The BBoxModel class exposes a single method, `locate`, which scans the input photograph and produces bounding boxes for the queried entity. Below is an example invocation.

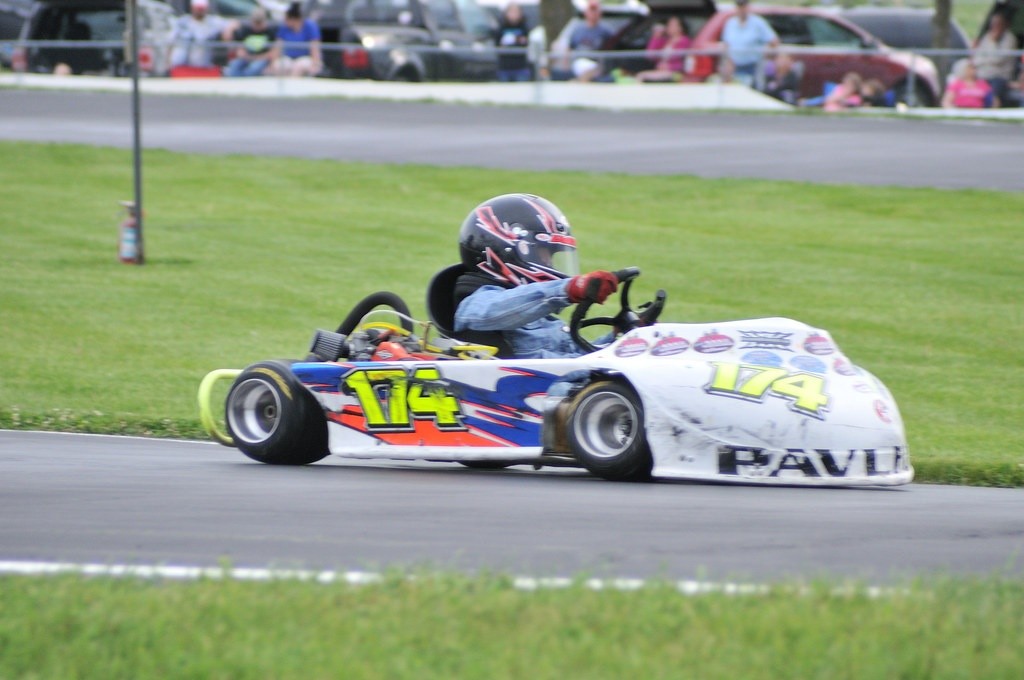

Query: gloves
[565,271,618,304]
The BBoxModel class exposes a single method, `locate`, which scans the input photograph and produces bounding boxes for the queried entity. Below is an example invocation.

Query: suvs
[842,9,973,88]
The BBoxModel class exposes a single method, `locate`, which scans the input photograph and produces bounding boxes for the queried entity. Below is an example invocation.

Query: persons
[223,11,283,76]
[822,71,898,112]
[938,15,1018,109]
[267,7,325,79]
[171,0,234,68]
[489,1,535,82]
[706,0,806,106]
[568,0,617,83]
[645,13,694,73]
[443,193,618,360]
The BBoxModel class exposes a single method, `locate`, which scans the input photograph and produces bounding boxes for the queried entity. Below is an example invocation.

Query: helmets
[458,193,580,287]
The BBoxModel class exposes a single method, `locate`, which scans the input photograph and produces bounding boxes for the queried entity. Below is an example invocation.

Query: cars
[549,0,718,82]
[0,0,273,76]
[319,0,503,83]
[687,5,944,106]
